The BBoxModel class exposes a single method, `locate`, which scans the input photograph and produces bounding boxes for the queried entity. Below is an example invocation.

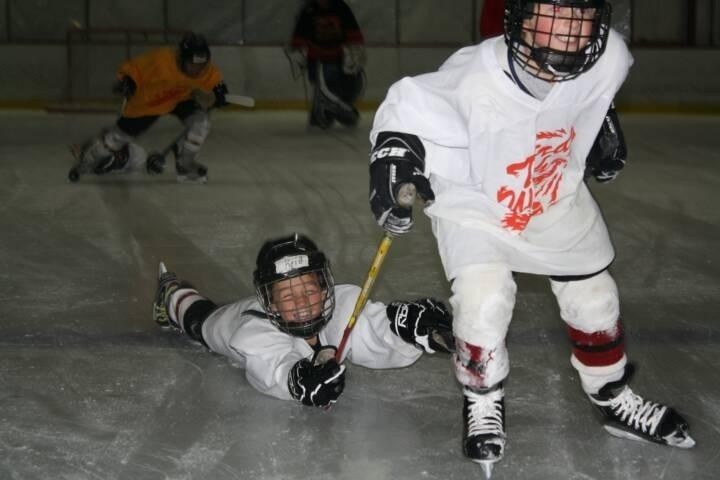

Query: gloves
[384,296,457,356]
[210,82,232,110]
[586,103,629,185]
[365,131,438,237]
[112,74,137,98]
[286,345,348,410]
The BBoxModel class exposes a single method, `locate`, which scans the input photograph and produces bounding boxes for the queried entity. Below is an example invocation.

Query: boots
[174,146,209,178]
[80,139,104,176]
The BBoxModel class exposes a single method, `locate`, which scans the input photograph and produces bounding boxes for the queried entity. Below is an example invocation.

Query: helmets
[249,230,333,309]
[176,29,212,70]
[501,0,611,61]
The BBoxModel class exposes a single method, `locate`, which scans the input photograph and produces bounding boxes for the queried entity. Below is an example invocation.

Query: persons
[69,32,229,185]
[364,0,693,467]
[281,0,369,131]
[151,230,454,410]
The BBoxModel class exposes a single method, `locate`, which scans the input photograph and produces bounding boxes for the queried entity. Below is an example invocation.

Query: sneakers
[582,362,691,447]
[458,373,510,462]
[152,271,180,328]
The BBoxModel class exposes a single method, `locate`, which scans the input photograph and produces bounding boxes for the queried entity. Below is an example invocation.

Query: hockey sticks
[335,184,415,363]
[161,93,255,159]
